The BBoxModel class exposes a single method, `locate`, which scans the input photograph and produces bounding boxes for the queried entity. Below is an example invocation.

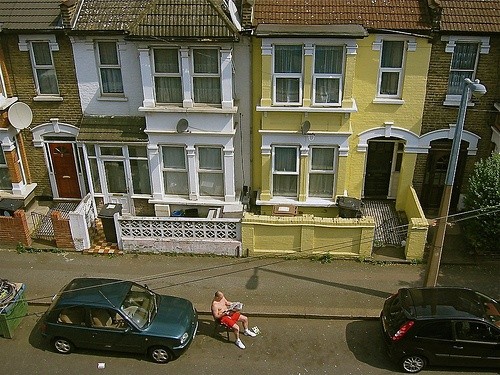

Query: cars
[38,277,200,365]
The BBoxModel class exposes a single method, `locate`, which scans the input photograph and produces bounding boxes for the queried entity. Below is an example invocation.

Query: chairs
[59,306,84,324]
[212,311,231,341]
[92,307,125,329]
[107,306,138,323]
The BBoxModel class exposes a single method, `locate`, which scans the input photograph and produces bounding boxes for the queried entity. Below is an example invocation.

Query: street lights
[422,77,487,288]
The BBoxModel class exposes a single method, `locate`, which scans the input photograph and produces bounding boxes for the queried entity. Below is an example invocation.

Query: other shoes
[235,340,246,349]
[244,330,256,337]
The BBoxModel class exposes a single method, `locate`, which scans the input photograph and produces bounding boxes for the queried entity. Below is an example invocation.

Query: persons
[211,291,257,350]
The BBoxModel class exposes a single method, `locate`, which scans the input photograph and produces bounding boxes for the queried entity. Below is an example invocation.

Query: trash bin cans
[336,196,364,218]
[0,198,24,217]
[97,202,123,244]
[272,204,298,217]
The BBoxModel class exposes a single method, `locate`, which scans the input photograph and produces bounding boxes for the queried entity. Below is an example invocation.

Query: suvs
[378,285,500,374]
